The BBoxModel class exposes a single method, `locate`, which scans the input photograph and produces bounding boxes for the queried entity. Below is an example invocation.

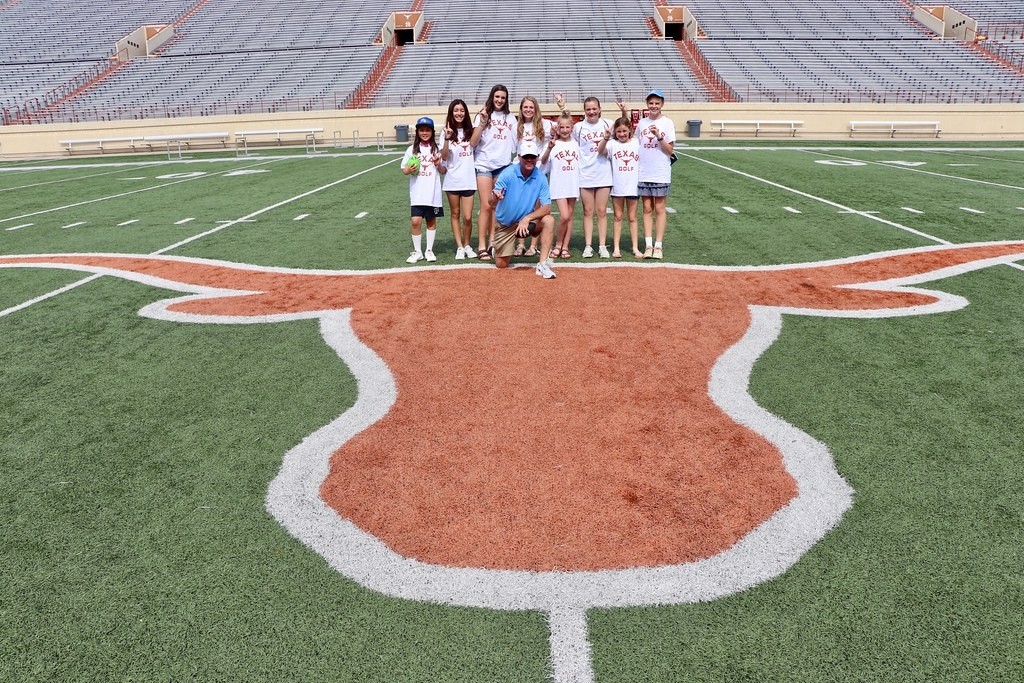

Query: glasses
[522,155,537,159]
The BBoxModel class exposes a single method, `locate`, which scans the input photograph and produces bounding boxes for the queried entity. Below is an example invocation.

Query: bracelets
[560,103,568,112]
[657,137,663,141]
[479,120,487,128]
[445,138,451,141]
[435,163,441,167]
[604,138,608,141]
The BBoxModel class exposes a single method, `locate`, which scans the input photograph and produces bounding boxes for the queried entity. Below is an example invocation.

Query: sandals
[549,247,572,259]
[514,244,525,256]
[477,246,493,261]
[524,247,538,257]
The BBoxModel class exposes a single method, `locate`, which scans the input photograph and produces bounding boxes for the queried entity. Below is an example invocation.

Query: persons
[550,96,628,258]
[541,114,581,258]
[634,90,676,259]
[512,91,569,256]
[487,141,558,279]
[400,117,448,264]
[436,99,478,260]
[597,116,675,259]
[470,84,519,261]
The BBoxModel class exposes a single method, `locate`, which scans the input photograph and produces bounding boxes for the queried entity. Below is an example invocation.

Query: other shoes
[633,250,643,259]
[612,251,621,257]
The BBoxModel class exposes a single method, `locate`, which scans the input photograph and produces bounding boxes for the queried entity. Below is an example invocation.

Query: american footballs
[515,222,536,238]
[408,155,420,175]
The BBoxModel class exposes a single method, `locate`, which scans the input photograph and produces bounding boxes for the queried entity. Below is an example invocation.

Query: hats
[415,117,434,128]
[518,141,538,157]
[645,89,664,99]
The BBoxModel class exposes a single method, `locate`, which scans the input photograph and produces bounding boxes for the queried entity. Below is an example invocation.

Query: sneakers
[405,250,424,264]
[598,246,610,259]
[424,250,436,262]
[535,261,556,279]
[582,246,594,258]
[643,246,663,260]
[454,245,477,259]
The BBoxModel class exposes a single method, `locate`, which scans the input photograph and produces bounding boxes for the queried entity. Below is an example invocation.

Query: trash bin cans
[687,120,702,137]
[394,124,409,142]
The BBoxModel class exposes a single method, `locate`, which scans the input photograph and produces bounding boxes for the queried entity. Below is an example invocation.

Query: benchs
[0,0,1024,157]
